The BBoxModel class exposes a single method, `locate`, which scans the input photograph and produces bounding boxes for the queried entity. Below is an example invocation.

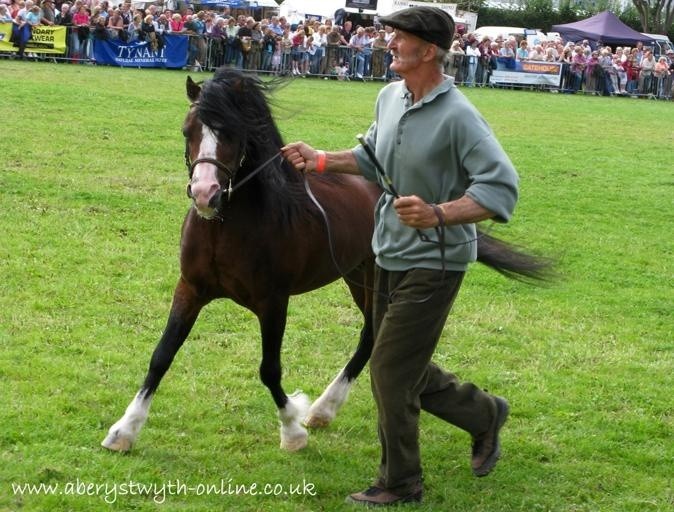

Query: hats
[378,7,456,51]
[665,50,673,55]
[568,41,574,47]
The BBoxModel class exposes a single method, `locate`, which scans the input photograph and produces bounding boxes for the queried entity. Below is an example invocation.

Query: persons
[280,6,521,505]
[0,0,674,99]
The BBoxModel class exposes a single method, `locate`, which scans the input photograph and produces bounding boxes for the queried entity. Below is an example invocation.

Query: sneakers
[292,68,300,76]
[471,393,507,477]
[615,88,627,94]
[345,485,423,504]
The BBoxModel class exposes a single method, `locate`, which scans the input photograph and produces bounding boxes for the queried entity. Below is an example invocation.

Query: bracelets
[315,149,325,174]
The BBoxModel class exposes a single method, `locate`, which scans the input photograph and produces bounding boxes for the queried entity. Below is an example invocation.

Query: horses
[100,61,558,455]
